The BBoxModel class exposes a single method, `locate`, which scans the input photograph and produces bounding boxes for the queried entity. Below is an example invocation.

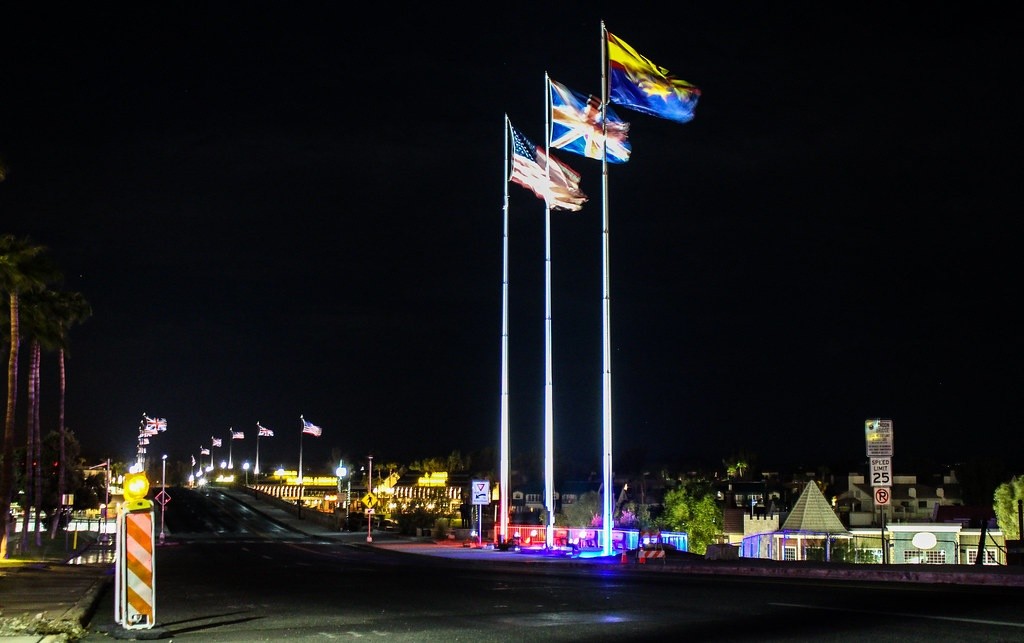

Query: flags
[191,457,196,466]
[550,78,631,164]
[258,425,274,436]
[213,438,222,447]
[302,418,322,436]
[508,118,589,212]
[201,449,210,455]
[607,31,700,124]
[138,417,166,463]
[232,432,244,439]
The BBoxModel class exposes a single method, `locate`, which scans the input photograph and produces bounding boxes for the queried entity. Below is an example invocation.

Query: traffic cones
[621,544,629,564]
[639,547,646,565]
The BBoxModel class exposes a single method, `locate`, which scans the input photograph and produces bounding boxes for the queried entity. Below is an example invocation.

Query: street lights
[243,462,250,486]
[278,468,285,499]
